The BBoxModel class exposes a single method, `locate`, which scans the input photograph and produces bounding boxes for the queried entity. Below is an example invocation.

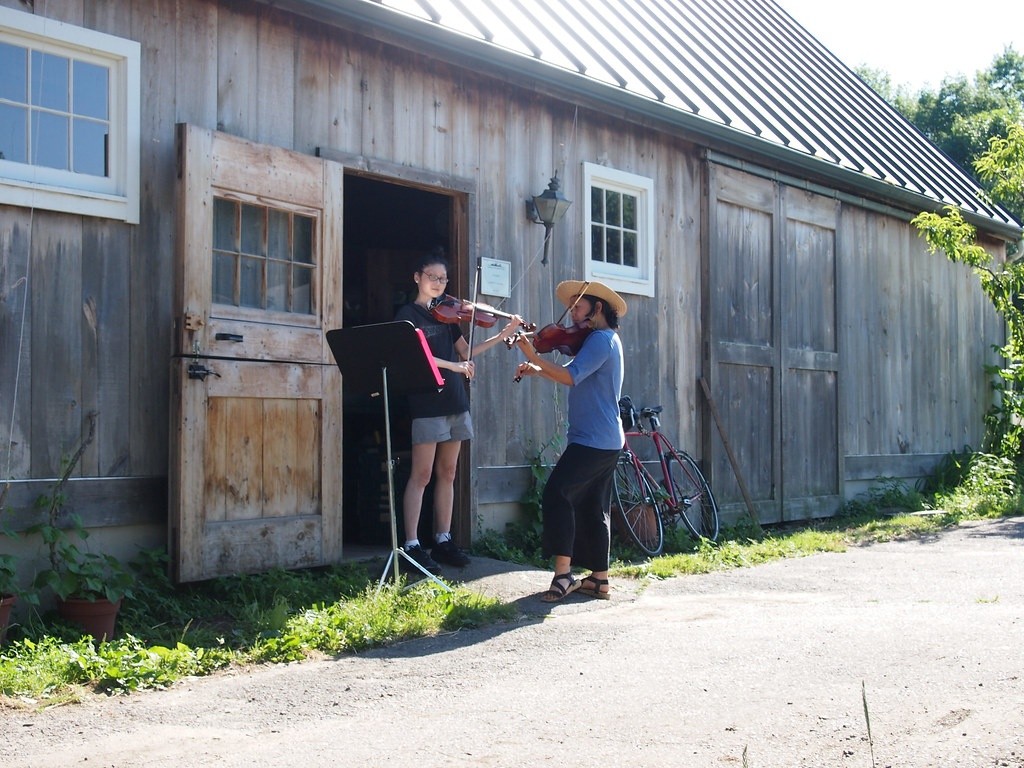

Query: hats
[556,280,627,318]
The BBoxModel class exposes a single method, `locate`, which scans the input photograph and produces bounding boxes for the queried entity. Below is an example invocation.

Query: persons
[514,279,627,605]
[392,253,524,571]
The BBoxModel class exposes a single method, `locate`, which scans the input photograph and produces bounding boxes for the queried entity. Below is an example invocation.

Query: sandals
[574,576,611,599]
[540,572,581,602]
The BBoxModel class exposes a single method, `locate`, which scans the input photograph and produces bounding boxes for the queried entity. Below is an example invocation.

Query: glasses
[421,270,449,284]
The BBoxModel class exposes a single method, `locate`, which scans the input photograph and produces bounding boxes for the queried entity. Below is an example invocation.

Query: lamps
[526,168,572,269]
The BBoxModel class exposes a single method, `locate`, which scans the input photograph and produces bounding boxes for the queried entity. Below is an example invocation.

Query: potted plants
[1,408,128,653]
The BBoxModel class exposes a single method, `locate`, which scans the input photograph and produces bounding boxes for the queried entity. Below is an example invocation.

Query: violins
[432,292,536,333]
[503,321,595,357]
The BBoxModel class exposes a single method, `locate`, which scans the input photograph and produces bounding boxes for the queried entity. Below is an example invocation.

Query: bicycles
[611,395,720,557]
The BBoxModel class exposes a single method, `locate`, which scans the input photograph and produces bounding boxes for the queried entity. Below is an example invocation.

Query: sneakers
[400,545,441,575]
[430,541,471,566]
